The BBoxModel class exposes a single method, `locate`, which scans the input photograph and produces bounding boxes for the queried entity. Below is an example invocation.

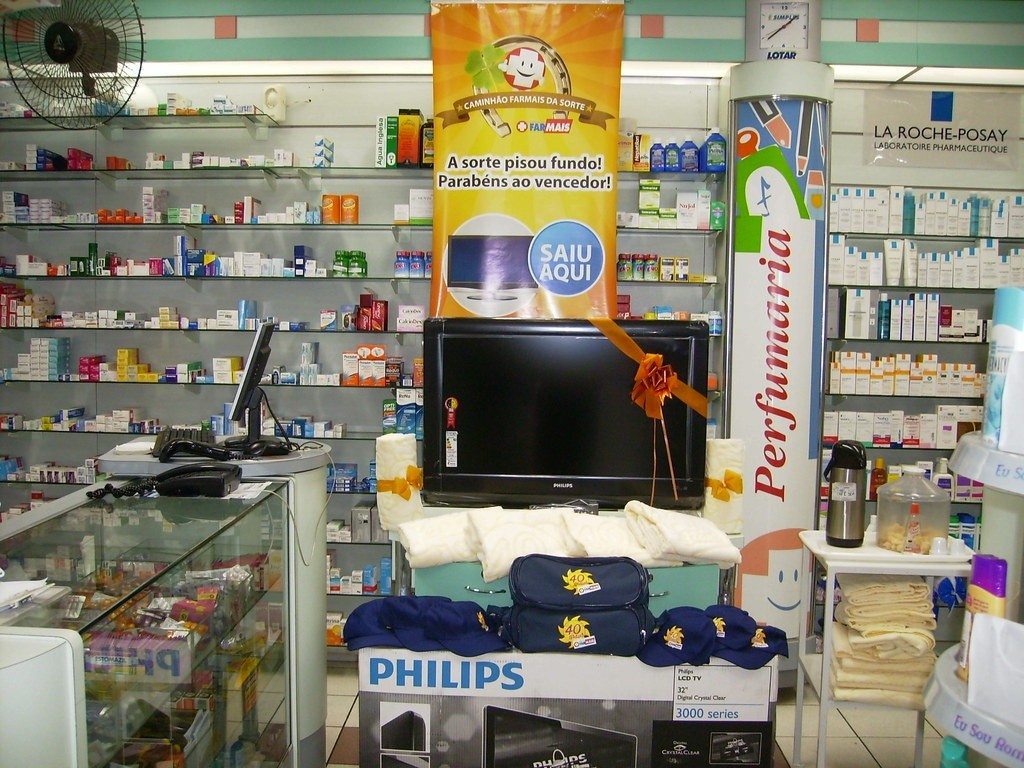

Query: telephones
[154,462,243,497]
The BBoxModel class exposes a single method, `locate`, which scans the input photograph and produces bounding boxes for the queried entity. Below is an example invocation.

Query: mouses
[243,441,289,457]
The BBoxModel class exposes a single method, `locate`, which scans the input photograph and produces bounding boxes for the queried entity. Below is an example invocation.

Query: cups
[929,537,965,556]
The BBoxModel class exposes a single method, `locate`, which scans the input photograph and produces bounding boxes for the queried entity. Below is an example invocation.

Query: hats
[705,604,757,651]
[636,606,715,667]
[711,626,790,670]
[343,598,404,651]
[425,600,512,657]
[381,595,452,652]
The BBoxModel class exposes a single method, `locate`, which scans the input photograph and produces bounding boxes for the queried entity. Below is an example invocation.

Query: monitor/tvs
[225,322,283,450]
[423,318,712,509]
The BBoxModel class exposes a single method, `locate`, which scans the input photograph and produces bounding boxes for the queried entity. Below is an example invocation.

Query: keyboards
[153,428,215,456]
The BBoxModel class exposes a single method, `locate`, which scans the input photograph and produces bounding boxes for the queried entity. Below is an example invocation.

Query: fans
[1,0,143,130]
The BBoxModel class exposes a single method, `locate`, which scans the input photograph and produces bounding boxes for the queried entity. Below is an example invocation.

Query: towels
[830,572,938,711]
[464,508,576,585]
[624,500,742,571]
[561,510,685,568]
[702,438,744,535]
[397,504,506,570]
[374,432,423,530]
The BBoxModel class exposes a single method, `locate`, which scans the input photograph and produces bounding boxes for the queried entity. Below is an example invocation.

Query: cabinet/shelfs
[1,112,436,662]
[793,527,978,767]
[822,230,1024,505]
[613,170,731,439]
[924,433,1024,768]
[1,477,295,767]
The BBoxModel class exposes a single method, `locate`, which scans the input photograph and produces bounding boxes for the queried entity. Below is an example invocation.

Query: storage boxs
[617,133,717,438]
[821,185,1024,500]
[2,105,434,645]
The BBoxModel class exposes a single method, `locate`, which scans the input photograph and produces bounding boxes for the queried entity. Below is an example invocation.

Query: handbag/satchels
[505,603,656,657]
[509,554,655,610]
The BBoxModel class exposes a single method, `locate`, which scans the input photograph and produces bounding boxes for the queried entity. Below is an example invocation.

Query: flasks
[823,439,867,549]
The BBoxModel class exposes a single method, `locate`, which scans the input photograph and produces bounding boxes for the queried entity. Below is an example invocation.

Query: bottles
[878,187,992,339]
[869,458,888,501]
[708,310,722,337]
[331,249,432,278]
[420,117,435,168]
[649,126,727,173]
[866,514,877,544]
[616,253,659,281]
[904,502,922,554]
[88,242,98,276]
[876,463,950,555]
[933,457,955,502]
